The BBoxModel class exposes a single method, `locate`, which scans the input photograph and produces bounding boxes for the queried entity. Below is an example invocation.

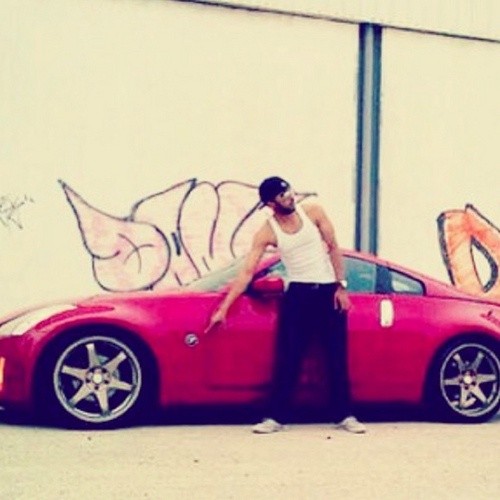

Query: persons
[204,176,366,434]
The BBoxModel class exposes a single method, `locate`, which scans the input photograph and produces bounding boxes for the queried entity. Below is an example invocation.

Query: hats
[258,176,289,206]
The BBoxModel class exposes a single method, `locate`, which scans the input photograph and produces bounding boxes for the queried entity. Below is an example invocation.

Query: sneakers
[252,418,282,433]
[337,416,366,433]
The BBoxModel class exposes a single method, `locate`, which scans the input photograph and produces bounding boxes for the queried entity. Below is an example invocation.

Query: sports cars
[0,240,500,429]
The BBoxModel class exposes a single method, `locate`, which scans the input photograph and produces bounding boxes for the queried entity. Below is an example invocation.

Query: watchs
[338,279,348,288]
